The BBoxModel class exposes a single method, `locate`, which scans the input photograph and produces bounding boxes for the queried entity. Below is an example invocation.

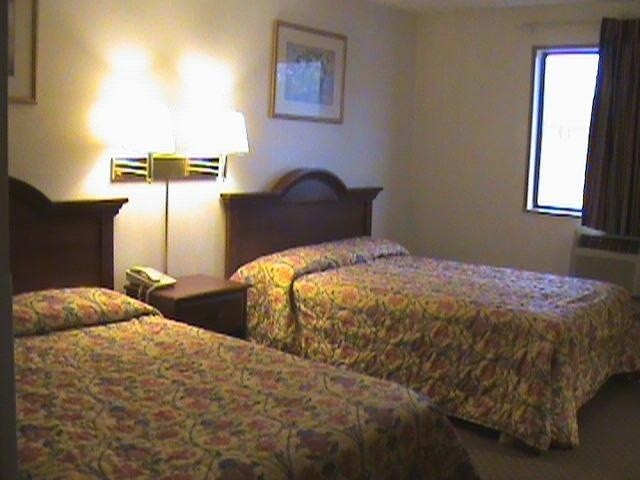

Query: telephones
[126,265,177,289]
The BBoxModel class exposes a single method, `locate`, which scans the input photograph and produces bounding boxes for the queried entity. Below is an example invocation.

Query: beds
[219,168,640,453]
[8,172,471,479]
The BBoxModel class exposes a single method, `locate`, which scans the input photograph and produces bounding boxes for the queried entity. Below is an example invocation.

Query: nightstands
[124,273,253,339]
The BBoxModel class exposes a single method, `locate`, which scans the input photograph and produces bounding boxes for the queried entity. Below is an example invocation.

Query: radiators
[568,227,640,299]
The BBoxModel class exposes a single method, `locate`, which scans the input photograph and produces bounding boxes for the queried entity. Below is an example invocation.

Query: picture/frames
[0,0,40,107]
[269,19,348,125]
